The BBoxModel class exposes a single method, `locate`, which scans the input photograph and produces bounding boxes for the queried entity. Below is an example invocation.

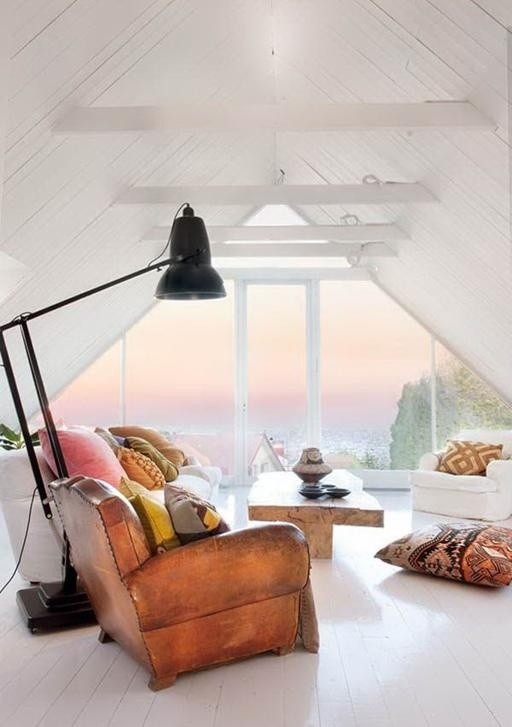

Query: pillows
[37,426,188,487]
[439,439,503,475]
[117,476,183,555]
[374,522,512,587]
[163,483,229,544]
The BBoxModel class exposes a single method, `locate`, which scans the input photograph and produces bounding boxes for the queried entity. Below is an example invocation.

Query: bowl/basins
[298,484,351,499]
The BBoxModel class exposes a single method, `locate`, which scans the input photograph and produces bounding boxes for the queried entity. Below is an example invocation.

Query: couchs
[0,431,222,584]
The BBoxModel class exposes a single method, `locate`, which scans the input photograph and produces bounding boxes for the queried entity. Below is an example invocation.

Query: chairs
[411,429,512,522]
[46,475,319,692]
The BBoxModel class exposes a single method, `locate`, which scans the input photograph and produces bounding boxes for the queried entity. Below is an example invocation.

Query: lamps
[0,201,226,631]
